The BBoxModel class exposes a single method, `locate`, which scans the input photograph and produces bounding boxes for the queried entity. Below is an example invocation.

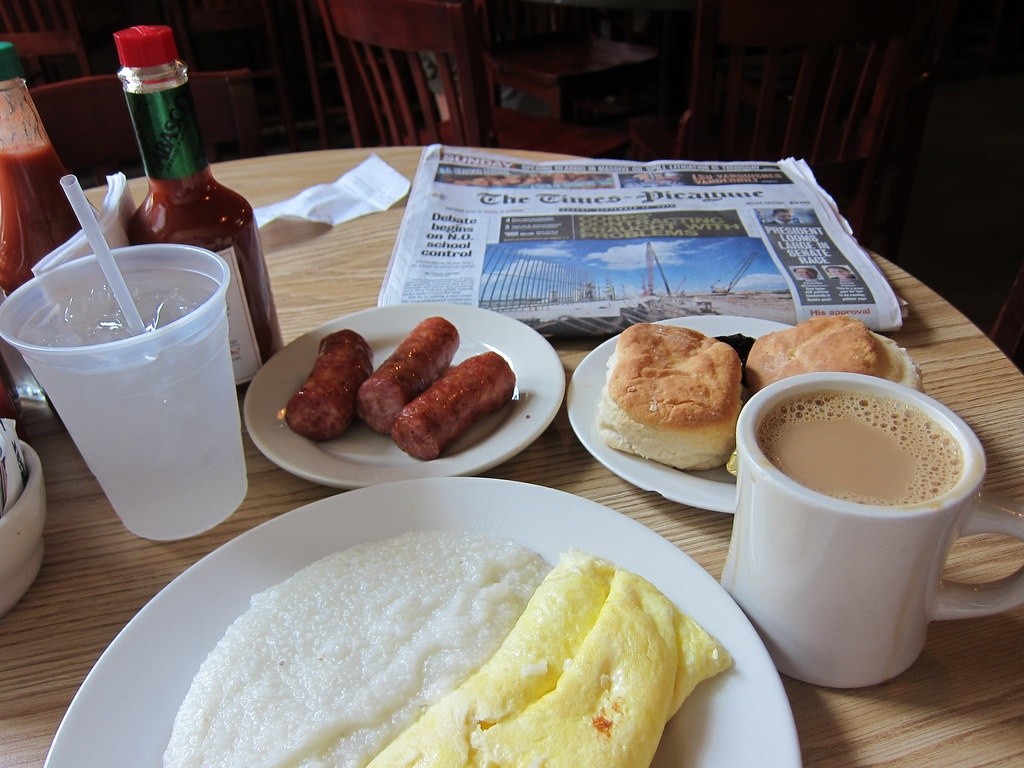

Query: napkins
[32,173,133,276]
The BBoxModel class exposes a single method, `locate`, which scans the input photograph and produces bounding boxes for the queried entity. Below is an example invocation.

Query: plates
[244,302,566,489]
[45,477,804,768]
[566,315,801,513]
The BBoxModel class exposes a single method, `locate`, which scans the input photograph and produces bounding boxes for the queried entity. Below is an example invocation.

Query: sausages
[284,329,374,443]
[354,316,461,434]
[392,351,516,460]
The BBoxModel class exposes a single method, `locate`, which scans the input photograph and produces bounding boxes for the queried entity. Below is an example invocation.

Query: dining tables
[0,149,1024,768]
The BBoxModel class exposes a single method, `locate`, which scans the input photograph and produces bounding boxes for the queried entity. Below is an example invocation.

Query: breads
[742,314,922,396]
[596,322,743,471]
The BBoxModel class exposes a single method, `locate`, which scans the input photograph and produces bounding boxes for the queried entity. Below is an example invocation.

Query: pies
[362,551,734,768]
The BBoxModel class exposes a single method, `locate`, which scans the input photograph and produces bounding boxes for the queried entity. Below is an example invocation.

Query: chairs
[0,0,946,266]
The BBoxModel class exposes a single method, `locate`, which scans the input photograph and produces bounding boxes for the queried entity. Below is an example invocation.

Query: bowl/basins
[0,438,47,618]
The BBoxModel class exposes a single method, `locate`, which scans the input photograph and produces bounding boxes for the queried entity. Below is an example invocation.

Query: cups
[1,242,250,540]
[720,373,1024,688]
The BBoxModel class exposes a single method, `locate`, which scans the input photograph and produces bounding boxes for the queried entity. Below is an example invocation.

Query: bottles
[0,41,107,423]
[114,25,284,399]
